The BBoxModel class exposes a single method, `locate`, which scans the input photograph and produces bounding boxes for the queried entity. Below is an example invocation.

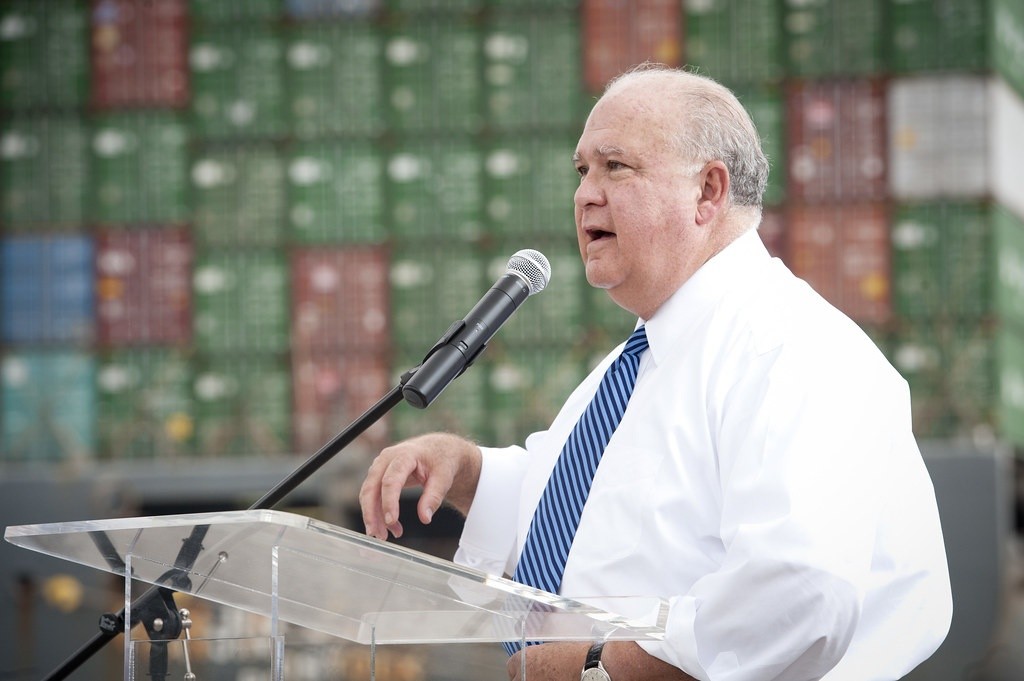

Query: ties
[501,327,649,658]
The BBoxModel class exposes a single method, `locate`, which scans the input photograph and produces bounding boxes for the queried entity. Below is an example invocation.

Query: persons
[359,61,958,680]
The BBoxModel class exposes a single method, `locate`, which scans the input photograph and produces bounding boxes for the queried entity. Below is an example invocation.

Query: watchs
[580,636,614,680]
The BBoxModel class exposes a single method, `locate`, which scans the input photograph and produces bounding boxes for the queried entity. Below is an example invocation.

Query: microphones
[400,249,553,409]
